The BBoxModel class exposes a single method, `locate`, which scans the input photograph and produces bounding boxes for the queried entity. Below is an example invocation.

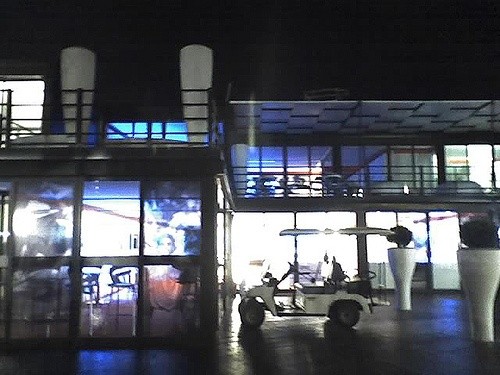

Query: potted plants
[458,218,500,343]
[386,225,417,311]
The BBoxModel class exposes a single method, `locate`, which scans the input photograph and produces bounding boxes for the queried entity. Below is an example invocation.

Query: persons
[320,254,346,282]
[260,256,270,278]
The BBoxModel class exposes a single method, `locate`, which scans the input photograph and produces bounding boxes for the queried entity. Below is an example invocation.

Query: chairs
[82,273,103,330]
[108,264,141,330]
